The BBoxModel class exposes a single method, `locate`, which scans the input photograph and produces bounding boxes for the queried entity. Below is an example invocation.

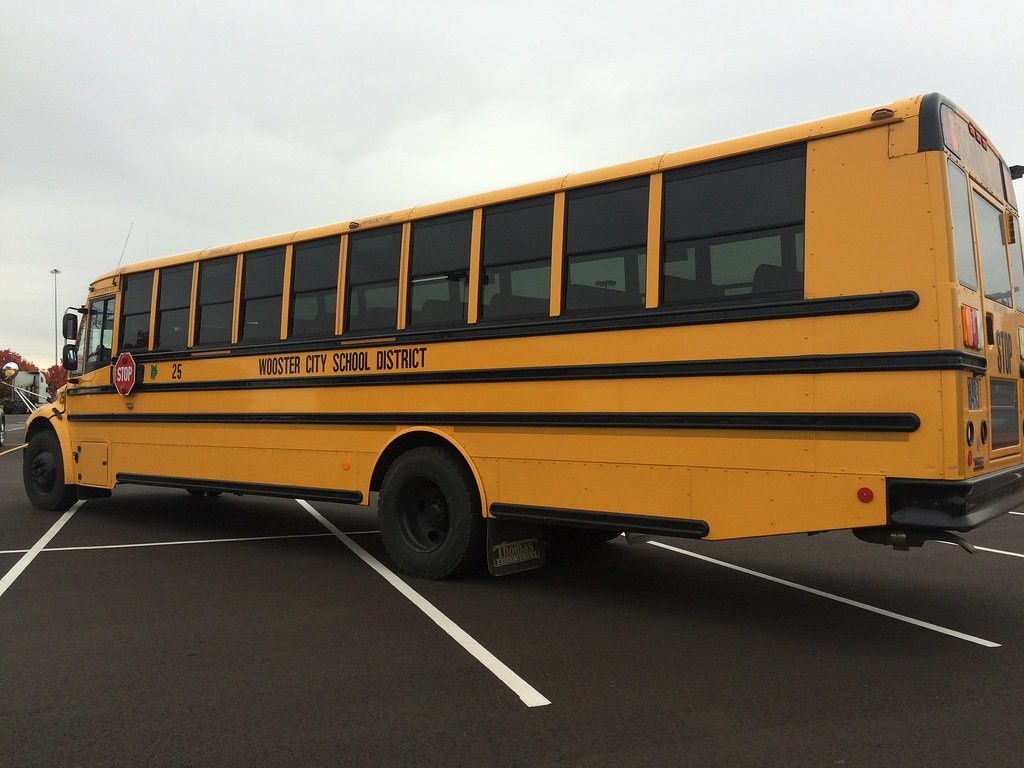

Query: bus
[0,93,1024,584]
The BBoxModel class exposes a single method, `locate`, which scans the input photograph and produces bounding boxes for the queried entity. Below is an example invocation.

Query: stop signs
[113,352,136,396]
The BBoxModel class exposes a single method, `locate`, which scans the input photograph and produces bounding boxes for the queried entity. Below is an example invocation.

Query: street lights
[595,279,616,289]
[50,269,61,367]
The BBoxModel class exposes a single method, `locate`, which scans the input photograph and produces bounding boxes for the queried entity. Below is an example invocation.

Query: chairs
[199,265,801,344]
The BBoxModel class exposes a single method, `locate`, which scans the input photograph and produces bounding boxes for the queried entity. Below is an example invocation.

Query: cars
[0,408,5,447]
